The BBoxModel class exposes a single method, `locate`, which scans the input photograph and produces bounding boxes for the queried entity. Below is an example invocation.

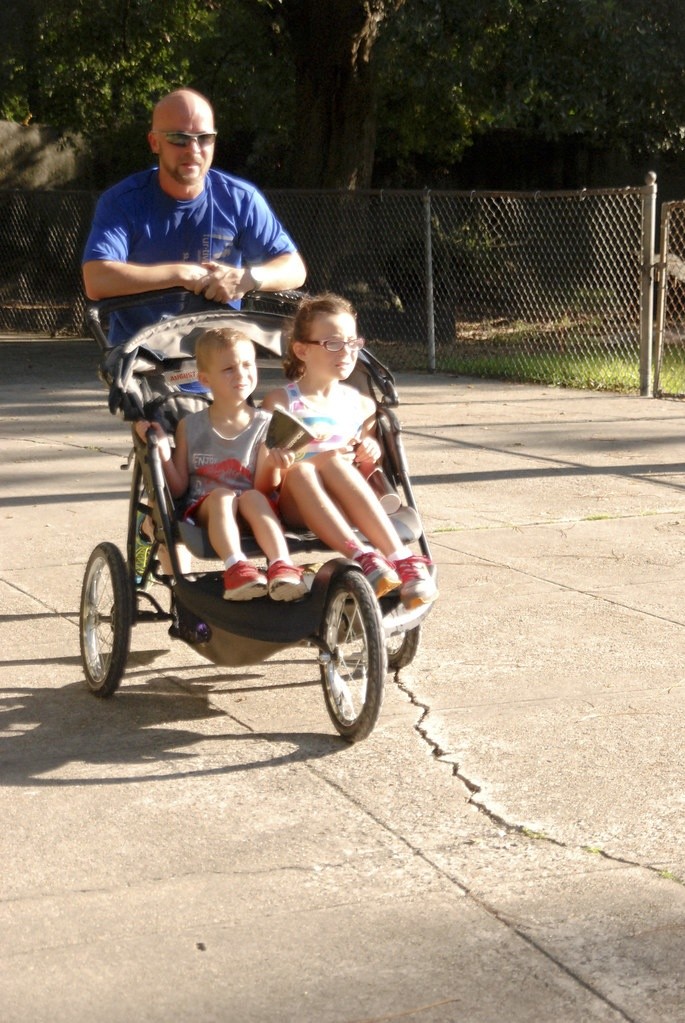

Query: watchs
[249,265,265,293]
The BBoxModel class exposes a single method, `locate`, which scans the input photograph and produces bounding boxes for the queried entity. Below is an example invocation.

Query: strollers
[78,289,432,744]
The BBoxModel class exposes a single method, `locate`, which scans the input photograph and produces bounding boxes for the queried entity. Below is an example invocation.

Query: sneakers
[266,560,309,603]
[221,560,268,601]
[134,515,153,584]
[345,541,402,599]
[392,555,439,610]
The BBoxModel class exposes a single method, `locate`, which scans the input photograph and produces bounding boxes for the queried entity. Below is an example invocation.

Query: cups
[366,468,401,515]
[264,405,317,453]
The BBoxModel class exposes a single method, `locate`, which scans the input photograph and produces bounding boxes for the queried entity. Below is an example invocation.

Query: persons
[81,89,307,642]
[261,299,439,610]
[135,328,309,602]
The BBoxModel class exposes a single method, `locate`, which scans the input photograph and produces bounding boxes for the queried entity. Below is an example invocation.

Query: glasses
[151,131,218,148]
[302,338,365,352]
